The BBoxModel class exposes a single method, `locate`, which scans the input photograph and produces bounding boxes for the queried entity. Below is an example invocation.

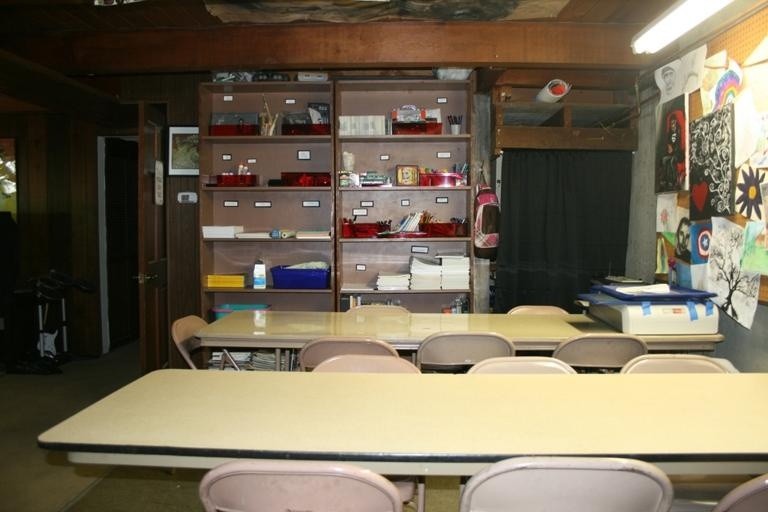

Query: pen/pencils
[377,219,391,225]
[341,215,358,224]
[447,113,463,125]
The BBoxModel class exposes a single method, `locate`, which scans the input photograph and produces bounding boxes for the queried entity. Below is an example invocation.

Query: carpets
[55,464,464,512]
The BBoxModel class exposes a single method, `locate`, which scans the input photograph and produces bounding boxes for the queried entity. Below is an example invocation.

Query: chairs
[351,302,407,311]
[169,314,220,368]
[301,338,398,372]
[508,305,570,315]
[558,337,647,376]
[310,353,419,374]
[626,354,738,376]
[417,333,516,377]
[463,356,572,374]
[714,474,768,512]
[200,461,404,512]
[461,455,676,512]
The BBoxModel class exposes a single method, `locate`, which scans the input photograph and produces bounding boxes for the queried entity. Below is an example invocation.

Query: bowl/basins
[436,68,473,80]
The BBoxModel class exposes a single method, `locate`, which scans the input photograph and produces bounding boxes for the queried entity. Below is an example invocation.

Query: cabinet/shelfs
[201,84,334,306]
[332,85,474,312]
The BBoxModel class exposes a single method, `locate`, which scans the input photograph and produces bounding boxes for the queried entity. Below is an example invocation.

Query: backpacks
[473,184,501,259]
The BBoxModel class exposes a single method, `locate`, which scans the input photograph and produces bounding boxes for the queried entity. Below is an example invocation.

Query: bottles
[441,294,470,314]
[216,75,235,83]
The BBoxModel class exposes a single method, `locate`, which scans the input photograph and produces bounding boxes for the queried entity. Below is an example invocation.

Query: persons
[676,218,691,263]
[660,67,676,98]
[654,115,684,190]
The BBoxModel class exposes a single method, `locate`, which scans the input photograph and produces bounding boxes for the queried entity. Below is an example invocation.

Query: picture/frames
[0,160,18,218]
[168,124,199,175]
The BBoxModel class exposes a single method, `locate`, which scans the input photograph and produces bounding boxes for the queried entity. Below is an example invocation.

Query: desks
[200,309,726,350]
[36,371,768,473]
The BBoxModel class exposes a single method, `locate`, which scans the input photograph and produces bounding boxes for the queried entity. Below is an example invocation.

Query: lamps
[630,1,741,55]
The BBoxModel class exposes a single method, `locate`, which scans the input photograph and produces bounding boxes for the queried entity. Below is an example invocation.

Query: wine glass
[253,310,266,335]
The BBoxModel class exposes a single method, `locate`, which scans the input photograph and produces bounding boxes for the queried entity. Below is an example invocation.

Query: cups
[339,179,353,187]
[342,223,352,238]
[451,124,462,135]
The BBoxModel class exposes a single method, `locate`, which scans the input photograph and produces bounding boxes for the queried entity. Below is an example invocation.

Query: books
[398,212,422,232]
[340,253,470,293]
[340,170,391,187]
[339,115,386,135]
[207,350,285,371]
[296,229,331,239]
[202,226,273,238]
